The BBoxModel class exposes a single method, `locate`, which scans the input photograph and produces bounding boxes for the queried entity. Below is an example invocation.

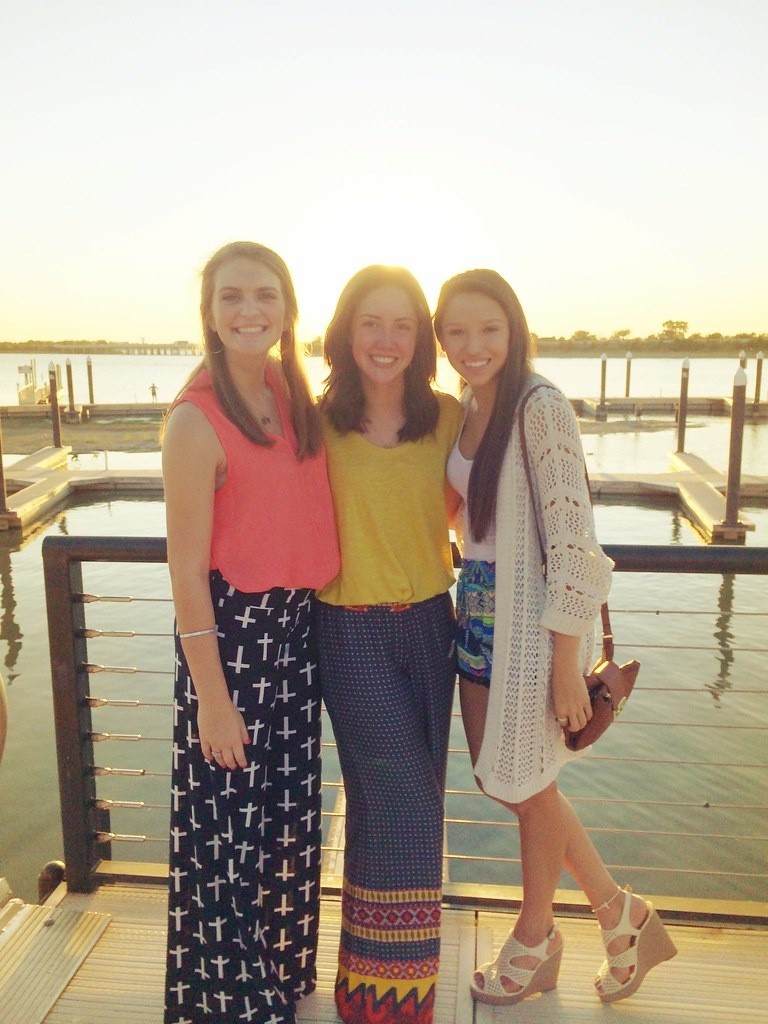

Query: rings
[558,717,567,721]
[212,752,221,758]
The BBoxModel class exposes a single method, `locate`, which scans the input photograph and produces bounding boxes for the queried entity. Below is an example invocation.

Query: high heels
[469,921,564,1006]
[593,883,678,1002]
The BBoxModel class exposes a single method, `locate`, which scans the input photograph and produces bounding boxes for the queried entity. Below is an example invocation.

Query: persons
[317,264,466,1024]
[149,384,158,402]
[433,269,677,1007]
[160,241,342,1024]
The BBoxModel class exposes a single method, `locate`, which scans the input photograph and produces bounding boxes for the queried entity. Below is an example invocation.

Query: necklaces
[244,397,272,426]
[366,417,406,448]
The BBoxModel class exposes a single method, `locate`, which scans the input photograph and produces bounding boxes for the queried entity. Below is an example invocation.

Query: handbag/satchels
[564,657,640,752]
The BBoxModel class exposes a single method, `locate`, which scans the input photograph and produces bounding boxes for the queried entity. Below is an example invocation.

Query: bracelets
[179,629,216,639]
